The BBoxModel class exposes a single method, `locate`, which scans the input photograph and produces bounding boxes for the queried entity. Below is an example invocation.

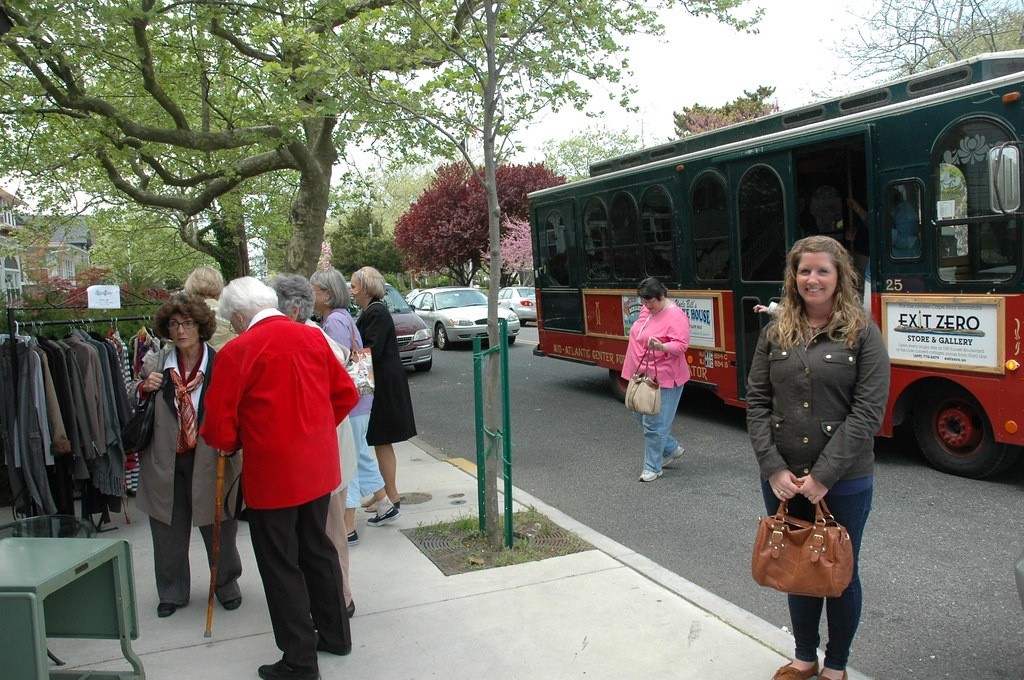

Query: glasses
[228,322,238,334]
[166,319,198,329]
[640,297,656,304]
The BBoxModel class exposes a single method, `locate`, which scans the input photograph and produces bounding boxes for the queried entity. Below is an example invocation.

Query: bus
[525,50,1024,481]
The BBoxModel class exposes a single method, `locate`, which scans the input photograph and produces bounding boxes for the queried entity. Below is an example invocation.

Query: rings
[156,383,158,386]
[780,492,784,495]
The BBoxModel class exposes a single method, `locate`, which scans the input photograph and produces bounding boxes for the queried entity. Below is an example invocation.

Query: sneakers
[661,446,685,468]
[638,470,663,482]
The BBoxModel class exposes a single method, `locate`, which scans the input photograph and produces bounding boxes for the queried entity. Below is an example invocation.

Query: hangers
[0,314,155,351]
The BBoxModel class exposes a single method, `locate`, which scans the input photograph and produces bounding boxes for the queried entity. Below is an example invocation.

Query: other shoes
[817,667,848,680]
[259,657,320,680]
[361,496,376,507]
[347,599,355,618]
[157,603,176,617]
[315,632,352,655]
[771,656,820,680]
[365,505,400,526]
[348,529,359,545]
[216,581,242,609]
[366,494,401,512]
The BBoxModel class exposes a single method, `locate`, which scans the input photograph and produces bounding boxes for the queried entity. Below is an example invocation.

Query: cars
[405,288,520,352]
[498,287,538,326]
[344,283,433,372]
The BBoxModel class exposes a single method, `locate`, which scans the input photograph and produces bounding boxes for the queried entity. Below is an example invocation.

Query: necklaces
[185,357,195,369]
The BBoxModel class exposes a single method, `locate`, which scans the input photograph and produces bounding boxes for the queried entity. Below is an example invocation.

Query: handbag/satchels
[120,395,157,455]
[751,484,854,598]
[342,349,375,397]
[625,347,660,414]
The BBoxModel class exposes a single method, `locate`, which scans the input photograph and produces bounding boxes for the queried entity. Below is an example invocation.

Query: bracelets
[855,205,861,214]
[662,344,663,350]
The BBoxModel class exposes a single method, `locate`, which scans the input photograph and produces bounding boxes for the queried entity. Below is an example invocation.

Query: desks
[0,537,146,680]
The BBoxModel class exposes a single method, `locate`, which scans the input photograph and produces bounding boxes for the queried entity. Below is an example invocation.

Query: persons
[748,237,889,680]
[312,270,399,546]
[350,266,417,512]
[201,275,352,680]
[847,188,920,320]
[182,265,238,350]
[621,277,690,482]
[264,273,356,616]
[128,293,244,617]
